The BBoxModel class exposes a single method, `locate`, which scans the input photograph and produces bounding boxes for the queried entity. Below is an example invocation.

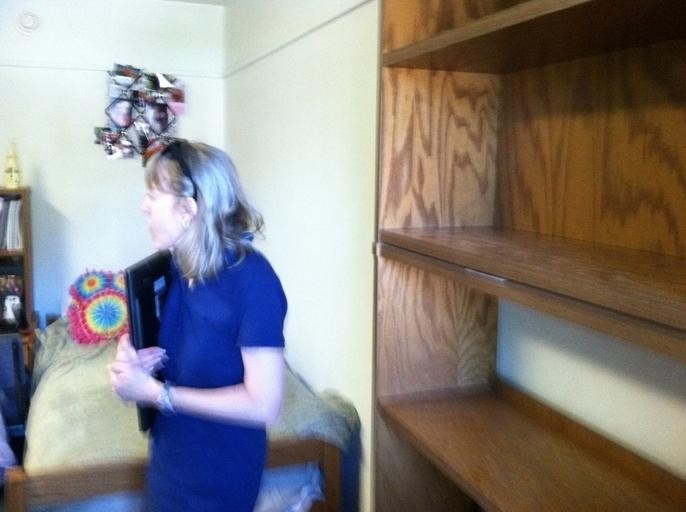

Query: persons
[107,141,289,512]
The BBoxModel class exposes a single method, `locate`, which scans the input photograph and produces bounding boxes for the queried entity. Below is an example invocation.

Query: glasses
[161,140,197,202]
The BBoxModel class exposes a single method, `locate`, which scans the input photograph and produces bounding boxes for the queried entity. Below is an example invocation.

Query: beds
[2,312,359,510]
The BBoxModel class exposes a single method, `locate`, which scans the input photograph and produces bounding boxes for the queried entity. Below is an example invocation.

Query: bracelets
[157,379,175,411]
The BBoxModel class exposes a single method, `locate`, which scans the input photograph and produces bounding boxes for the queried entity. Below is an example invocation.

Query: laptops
[125,247,172,432]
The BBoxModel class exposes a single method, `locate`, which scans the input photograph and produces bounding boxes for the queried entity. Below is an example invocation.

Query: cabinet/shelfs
[0,187,33,378]
[370,0,686,511]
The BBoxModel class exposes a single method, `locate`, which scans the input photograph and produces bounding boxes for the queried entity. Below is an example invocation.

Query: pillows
[69,267,131,346]
